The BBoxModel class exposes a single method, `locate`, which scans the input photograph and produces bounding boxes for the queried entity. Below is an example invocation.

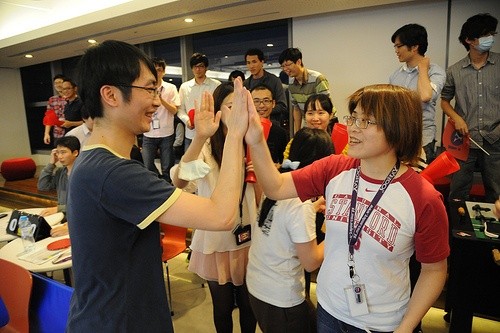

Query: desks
[432,197,500,318]
[0,208,71,272]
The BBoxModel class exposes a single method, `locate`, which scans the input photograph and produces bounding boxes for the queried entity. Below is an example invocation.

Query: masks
[470,35,495,52]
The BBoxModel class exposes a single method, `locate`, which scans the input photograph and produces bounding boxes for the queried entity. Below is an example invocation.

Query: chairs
[0,258,34,333]
[158,221,207,317]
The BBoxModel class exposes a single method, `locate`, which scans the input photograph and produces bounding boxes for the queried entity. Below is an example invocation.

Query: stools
[1,157,38,182]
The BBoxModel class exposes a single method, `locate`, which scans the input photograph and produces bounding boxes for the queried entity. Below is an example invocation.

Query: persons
[67,40,248,333]
[35,13,499,240]
[245,84,449,333]
[245,128,333,333]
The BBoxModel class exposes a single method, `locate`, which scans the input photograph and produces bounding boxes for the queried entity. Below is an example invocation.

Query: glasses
[394,43,409,50]
[253,98,274,105]
[477,32,498,37]
[342,115,377,129]
[114,85,163,100]
[55,81,64,85]
[157,68,164,74]
[63,87,75,91]
[193,64,205,69]
[280,62,296,71]
